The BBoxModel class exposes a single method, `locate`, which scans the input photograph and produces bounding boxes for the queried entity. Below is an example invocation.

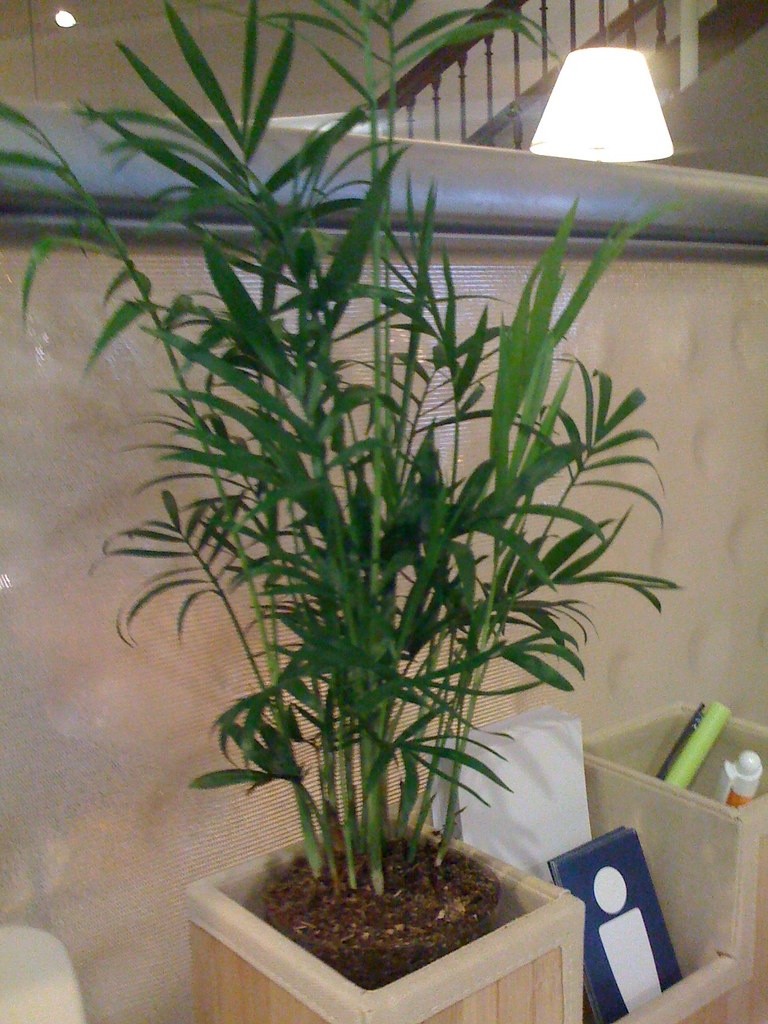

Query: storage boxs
[575,702,768,1024]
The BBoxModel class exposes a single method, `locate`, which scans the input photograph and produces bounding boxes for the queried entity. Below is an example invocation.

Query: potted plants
[0,0,699,1024]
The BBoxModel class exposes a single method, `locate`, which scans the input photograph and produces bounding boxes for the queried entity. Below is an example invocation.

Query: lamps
[528,0,676,166]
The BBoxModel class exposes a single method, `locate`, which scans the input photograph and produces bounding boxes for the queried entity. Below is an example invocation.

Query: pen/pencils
[655,699,732,789]
[717,749,764,806]
